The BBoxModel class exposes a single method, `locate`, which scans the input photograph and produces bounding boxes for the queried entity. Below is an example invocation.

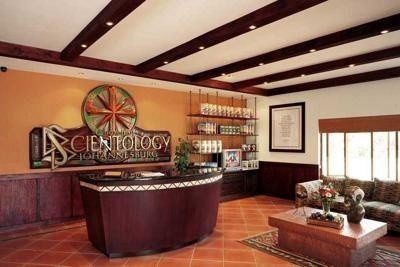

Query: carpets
[238,228,400,267]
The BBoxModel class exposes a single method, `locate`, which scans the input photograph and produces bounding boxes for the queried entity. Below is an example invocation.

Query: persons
[343,185,366,224]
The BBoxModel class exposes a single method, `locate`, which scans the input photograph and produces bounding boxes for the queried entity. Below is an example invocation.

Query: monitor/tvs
[212,149,243,173]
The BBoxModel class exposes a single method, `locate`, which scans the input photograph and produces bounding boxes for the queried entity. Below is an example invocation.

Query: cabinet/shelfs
[187,89,260,202]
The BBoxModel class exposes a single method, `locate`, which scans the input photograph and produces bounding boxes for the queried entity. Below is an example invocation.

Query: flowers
[311,182,339,212]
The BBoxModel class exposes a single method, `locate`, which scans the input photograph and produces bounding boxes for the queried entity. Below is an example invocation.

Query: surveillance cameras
[0,65,8,73]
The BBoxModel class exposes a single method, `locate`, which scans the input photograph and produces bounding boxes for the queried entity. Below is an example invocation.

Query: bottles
[201,139,223,154]
[220,123,241,135]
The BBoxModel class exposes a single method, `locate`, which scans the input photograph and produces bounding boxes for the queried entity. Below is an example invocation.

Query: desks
[268,207,387,267]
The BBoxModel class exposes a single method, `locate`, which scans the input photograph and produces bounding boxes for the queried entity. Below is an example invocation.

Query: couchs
[294,179,400,236]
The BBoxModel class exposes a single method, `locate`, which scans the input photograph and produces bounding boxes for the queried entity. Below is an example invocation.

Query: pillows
[344,178,374,201]
[322,176,346,196]
[371,178,400,205]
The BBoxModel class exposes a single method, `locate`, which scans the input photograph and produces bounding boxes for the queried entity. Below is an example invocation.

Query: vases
[322,201,330,216]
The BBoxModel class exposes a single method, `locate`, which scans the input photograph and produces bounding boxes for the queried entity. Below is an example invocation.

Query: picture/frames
[269,102,305,152]
